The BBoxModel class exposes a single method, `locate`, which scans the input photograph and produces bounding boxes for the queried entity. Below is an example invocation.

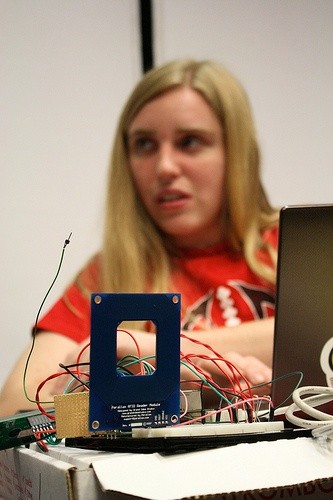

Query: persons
[0,58,280,419]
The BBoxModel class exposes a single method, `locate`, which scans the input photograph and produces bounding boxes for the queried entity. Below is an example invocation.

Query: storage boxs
[0,437,333,499]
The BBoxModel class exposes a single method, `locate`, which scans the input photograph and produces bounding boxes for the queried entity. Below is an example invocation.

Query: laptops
[267,203,333,424]
